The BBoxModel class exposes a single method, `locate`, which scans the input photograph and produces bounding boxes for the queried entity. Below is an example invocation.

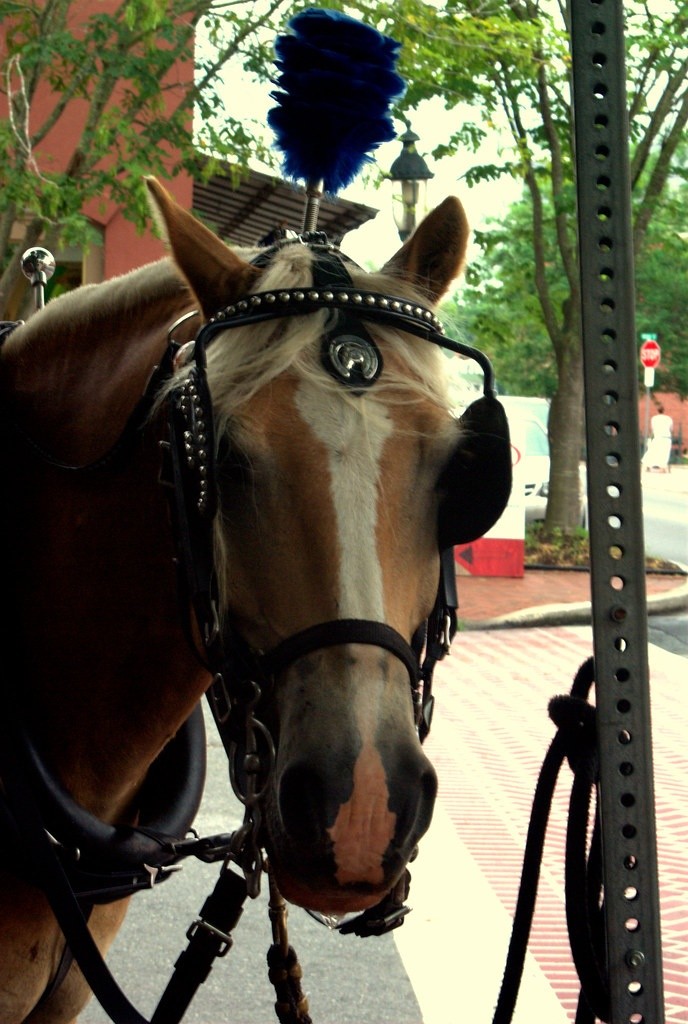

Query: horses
[0,172,511,1024]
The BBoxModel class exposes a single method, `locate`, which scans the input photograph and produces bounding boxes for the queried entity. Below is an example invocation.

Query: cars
[639,340,662,369]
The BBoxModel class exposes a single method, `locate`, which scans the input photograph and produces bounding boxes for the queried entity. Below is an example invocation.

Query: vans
[494,397,591,535]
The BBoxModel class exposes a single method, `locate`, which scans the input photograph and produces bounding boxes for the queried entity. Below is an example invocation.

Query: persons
[640,407,673,474]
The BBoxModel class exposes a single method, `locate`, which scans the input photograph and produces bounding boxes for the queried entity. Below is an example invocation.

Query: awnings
[191,147,379,249]
